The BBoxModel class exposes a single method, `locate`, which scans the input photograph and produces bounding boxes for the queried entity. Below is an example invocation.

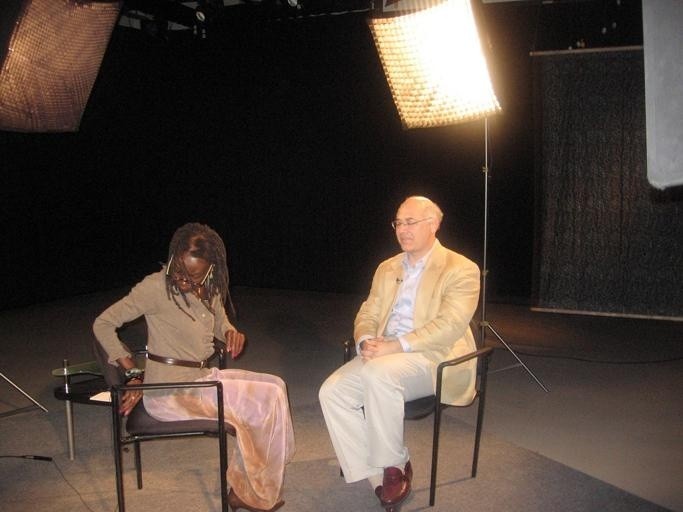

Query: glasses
[391,217,432,230]
[165,253,213,288]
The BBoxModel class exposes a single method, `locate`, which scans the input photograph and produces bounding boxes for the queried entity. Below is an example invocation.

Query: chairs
[92,319,236,511]
[340,337,494,506]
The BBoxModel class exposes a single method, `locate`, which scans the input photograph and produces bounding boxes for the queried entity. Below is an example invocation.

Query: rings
[131,396,135,399]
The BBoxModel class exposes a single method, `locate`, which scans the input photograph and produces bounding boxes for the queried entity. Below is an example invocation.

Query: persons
[315,195,483,512]
[90,224,294,511]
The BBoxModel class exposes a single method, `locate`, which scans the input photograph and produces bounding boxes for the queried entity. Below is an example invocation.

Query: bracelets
[121,366,143,383]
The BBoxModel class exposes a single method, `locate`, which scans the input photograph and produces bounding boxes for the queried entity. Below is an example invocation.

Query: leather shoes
[375,460,413,512]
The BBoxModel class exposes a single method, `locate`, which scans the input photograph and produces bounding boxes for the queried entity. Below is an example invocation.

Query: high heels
[227,486,285,512]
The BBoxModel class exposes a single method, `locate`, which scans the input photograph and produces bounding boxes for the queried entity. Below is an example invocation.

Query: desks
[52,357,104,461]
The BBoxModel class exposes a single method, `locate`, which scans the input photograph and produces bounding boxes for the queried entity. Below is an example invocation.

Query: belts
[146,350,219,369]
